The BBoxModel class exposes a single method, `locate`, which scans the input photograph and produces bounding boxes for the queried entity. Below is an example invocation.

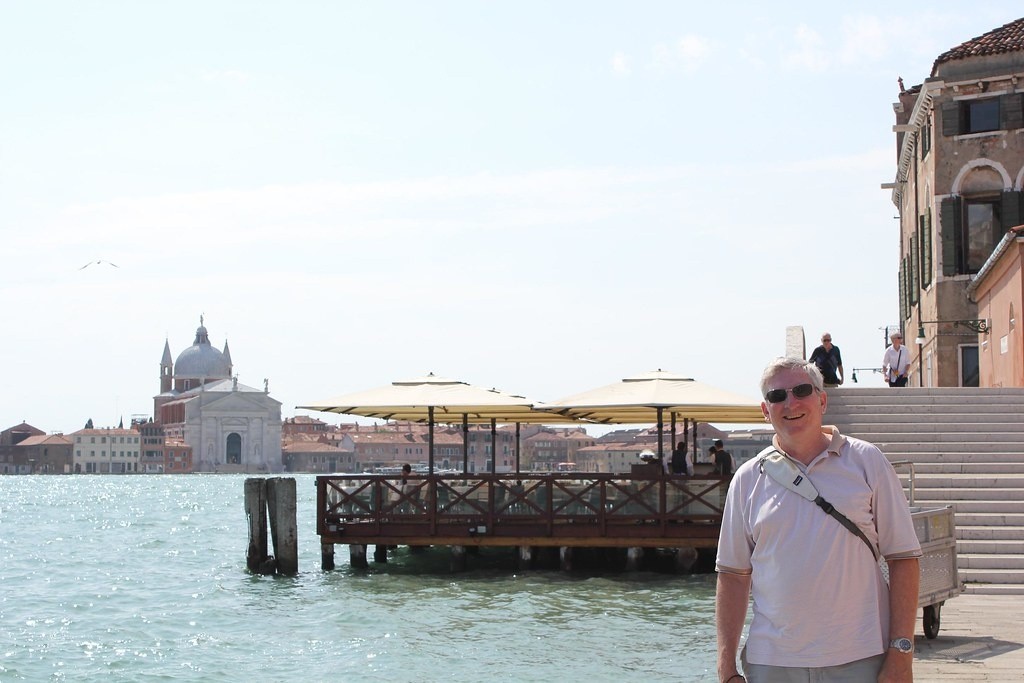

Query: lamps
[977,81,986,93]
[915,319,992,344]
[953,85,960,93]
[1012,76,1018,85]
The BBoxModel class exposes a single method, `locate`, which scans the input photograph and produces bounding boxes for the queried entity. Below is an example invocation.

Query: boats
[363,463,440,474]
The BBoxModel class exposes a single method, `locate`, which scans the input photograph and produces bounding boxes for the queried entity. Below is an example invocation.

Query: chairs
[335,478,647,523]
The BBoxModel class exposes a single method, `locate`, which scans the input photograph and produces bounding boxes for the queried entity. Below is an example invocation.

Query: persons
[515,481,527,511]
[808,332,844,388]
[709,446,716,455]
[715,358,924,683]
[665,442,694,523]
[883,333,911,387]
[394,463,424,514]
[712,440,735,473]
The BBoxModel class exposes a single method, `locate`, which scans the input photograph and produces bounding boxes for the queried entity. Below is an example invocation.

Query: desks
[451,486,489,511]
[553,484,589,498]
[340,485,403,521]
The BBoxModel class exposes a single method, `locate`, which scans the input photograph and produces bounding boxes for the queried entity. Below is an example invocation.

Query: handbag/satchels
[896,375,907,387]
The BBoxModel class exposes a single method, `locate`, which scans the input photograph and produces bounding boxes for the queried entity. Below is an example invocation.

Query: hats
[403,464,411,473]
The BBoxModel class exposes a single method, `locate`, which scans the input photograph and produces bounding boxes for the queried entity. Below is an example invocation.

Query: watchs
[889,638,914,653]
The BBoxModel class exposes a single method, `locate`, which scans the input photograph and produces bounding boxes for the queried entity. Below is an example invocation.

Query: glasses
[895,337,902,339]
[823,339,830,342]
[764,384,820,403]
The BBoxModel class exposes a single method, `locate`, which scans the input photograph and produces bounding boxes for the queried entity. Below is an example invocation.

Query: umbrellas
[296,372,771,473]
